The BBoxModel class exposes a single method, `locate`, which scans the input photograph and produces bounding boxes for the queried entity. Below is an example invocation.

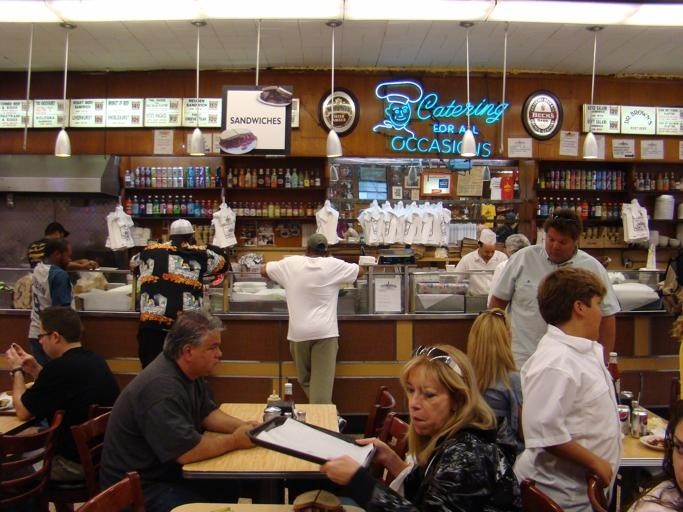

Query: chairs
[0,379,682,511]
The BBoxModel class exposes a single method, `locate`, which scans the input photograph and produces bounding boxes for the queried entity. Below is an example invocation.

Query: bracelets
[9,367,25,376]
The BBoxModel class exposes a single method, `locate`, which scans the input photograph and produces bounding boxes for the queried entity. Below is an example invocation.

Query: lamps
[581,26,602,162]
[187,20,207,156]
[458,21,478,157]
[52,23,77,159]
[322,19,343,159]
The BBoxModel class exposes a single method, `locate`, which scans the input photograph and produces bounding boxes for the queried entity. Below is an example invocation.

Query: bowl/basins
[642,227,683,248]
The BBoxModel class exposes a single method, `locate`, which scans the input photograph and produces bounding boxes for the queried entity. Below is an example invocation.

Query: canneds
[617,405,631,434]
[631,408,648,437]
[620,391,635,405]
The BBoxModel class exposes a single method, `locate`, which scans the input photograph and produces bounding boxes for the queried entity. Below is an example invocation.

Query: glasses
[551,215,577,225]
[479,310,506,326]
[415,344,464,377]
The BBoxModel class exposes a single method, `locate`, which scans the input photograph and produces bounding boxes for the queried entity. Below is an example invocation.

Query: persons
[129,217,230,369]
[27,221,100,270]
[5,305,120,485]
[98,310,260,512]
[260,234,366,433]
[466,307,526,463]
[456,229,508,295]
[28,240,76,367]
[487,233,531,308]
[320,344,523,512]
[490,209,622,374]
[627,400,683,512]
[512,268,623,512]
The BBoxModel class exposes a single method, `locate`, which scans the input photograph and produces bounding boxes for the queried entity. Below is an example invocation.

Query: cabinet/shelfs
[116,154,363,252]
[518,156,682,271]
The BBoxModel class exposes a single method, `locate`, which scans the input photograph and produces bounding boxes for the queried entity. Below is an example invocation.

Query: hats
[480,229,496,245]
[170,219,195,234]
[308,234,329,251]
[45,222,71,238]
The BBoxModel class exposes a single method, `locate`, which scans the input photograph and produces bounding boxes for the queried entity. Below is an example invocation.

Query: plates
[678,203,683,219]
[232,281,266,292]
[653,195,674,220]
[637,268,658,288]
[637,432,668,452]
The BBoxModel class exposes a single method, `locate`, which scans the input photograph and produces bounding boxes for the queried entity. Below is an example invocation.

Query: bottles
[123,169,134,188]
[605,350,619,398]
[124,195,323,217]
[263,382,305,423]
[633,170,683,190]
[226,164,320,188]
[533,196,626,220]
